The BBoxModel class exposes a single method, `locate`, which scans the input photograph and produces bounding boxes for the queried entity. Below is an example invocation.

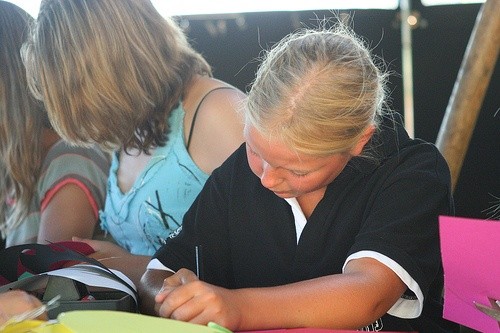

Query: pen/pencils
[196,245,204,281]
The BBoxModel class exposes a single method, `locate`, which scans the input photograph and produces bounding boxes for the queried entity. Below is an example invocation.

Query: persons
[0,0,112,304]
[20,1,249,285]
[136,10,460,333]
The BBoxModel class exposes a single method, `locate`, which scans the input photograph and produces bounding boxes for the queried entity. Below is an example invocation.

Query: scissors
[473,298,500,325]
